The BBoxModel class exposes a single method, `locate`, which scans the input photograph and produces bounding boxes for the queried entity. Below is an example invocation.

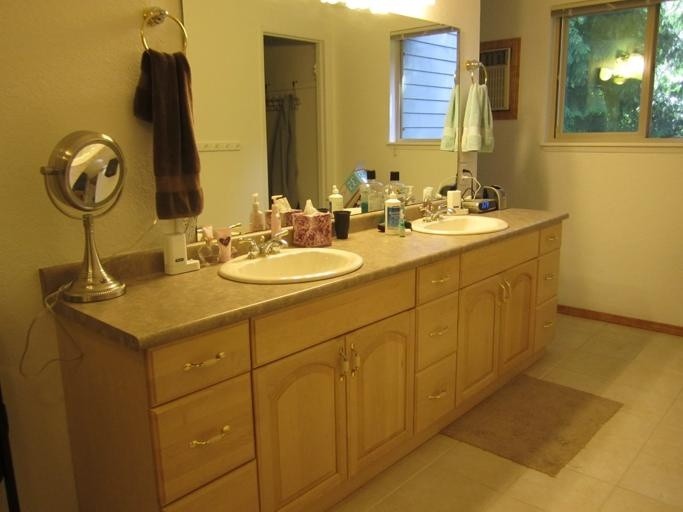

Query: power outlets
[172,217,192,233]
[457,159,471,188]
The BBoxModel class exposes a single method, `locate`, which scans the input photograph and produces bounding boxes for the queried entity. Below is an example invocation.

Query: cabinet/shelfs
[452,226,539,411]
[249,261,418,512]
[534,211,569,358]
[410,248,462,446]
[52,306,260,512]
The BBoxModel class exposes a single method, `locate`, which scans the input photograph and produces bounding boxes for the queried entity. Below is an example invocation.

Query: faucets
[432,209,456,221]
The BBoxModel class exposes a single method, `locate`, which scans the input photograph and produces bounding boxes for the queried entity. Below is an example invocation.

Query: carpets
[434,370,625,479]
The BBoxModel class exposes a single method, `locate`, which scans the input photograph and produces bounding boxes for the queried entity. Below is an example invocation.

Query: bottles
[359,169,384,213]
[383,170,406,211]
[399,210,406,237]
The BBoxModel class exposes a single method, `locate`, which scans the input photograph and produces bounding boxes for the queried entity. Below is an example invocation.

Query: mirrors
[178,0,462,247]
[39,130,133,306]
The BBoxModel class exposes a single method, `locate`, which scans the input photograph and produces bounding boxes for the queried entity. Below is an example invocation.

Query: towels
[460,84,495,153]
[438,82,460,153]
[267,94,300,209]
[130,45,205,230]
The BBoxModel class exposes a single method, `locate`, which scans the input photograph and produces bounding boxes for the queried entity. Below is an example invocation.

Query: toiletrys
[272,204,282,237]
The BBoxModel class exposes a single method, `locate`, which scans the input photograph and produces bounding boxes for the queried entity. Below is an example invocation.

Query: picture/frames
[479,37,522,121]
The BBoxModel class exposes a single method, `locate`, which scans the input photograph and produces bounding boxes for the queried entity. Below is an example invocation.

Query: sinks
[412,213,509,235]
[218,246,362,284]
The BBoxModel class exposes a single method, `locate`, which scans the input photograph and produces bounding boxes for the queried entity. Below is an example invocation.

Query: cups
[366,191,385,213]
[332,209,352,239]
